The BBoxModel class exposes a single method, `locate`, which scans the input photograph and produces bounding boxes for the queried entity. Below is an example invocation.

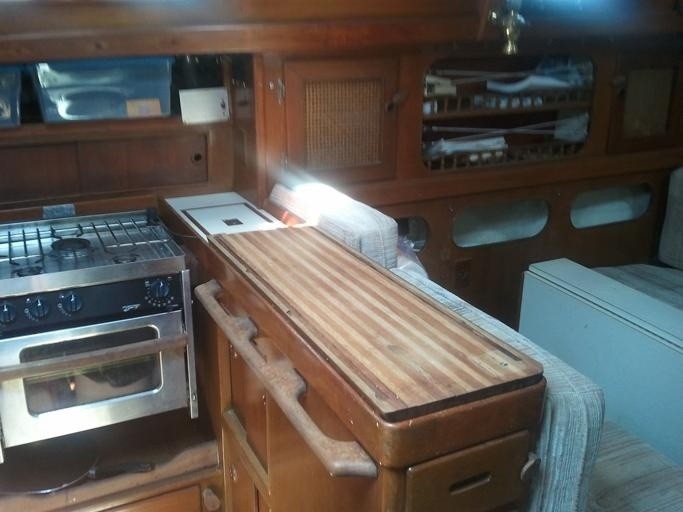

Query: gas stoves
[0,206,187,299]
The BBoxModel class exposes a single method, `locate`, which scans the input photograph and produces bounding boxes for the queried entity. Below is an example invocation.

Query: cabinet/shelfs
[227,26,683,337]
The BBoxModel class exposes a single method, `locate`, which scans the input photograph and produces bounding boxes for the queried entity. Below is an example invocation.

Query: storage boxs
[0,55,175,130]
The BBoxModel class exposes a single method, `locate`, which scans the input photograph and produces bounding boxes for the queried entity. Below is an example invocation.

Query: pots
[0,429,153,498]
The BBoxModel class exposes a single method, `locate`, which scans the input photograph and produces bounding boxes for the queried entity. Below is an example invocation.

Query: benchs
[268,169,682,511]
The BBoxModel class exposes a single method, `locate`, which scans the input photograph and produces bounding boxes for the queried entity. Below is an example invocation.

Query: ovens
[0,270,200,450]
